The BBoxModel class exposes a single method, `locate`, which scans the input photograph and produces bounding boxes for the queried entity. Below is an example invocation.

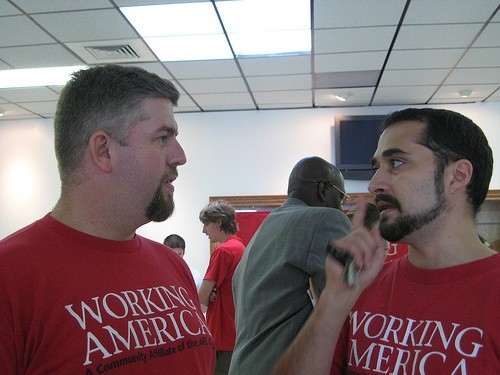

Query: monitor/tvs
[335,115,385,169]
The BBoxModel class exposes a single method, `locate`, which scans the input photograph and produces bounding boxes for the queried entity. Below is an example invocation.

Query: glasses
[315,181,351,205]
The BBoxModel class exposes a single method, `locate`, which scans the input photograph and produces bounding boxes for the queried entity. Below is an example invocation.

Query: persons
[0,65,216,375]
[198,200,247,375]
[163,234,185,258]
[272,108,500,375]
[228,156,355,375]
